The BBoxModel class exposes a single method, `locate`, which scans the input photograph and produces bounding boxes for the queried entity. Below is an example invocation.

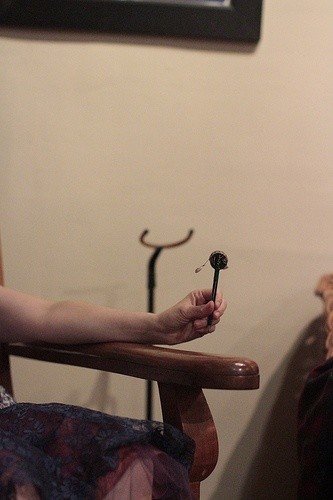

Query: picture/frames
[1,0,262,44]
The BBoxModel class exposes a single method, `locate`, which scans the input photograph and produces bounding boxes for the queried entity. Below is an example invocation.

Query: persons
[0,283,227,499]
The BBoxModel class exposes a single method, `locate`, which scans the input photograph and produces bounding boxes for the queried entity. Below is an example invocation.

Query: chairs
[0,248,261,500]
